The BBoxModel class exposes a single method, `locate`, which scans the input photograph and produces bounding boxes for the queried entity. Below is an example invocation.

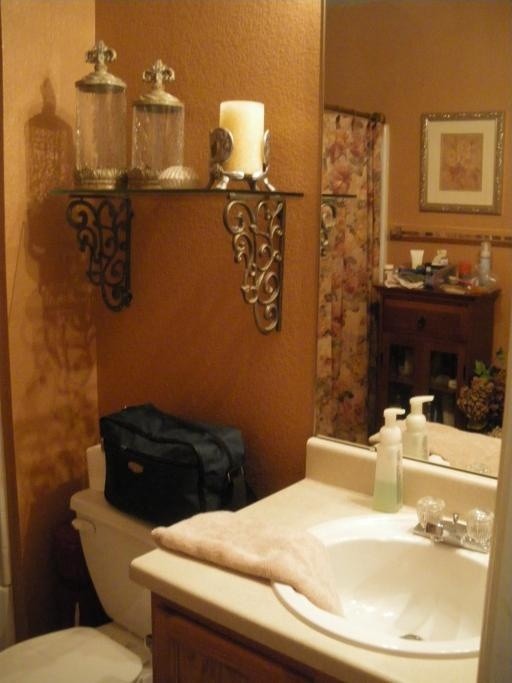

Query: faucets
[426,512,466,547]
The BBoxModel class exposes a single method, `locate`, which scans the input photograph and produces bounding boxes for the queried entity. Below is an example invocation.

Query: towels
[148,506,344,615]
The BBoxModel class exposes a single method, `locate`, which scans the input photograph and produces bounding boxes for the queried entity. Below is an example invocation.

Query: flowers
[453,364,505,422]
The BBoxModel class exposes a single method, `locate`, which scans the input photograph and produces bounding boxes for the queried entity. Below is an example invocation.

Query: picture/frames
[418,109,505,214]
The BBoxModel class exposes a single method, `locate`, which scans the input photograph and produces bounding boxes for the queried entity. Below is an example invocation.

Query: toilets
[0,488,161,683]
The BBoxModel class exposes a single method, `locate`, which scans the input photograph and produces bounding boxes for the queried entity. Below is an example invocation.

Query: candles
[216,99,265,177]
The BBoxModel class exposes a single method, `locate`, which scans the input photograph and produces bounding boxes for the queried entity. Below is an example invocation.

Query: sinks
[270,512,492,657]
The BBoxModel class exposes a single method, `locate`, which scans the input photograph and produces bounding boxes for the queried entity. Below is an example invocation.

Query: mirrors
[304,0,512,484]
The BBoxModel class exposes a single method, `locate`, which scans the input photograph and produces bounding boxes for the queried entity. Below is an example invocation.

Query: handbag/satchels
[99,403,258,526]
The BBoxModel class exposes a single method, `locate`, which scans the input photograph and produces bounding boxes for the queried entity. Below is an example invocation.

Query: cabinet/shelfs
[367,281,503,430]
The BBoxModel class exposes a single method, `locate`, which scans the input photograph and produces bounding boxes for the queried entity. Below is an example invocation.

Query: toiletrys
[479,240,490,280]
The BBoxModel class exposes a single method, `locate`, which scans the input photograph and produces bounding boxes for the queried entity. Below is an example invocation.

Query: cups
[410,249,425,270]
